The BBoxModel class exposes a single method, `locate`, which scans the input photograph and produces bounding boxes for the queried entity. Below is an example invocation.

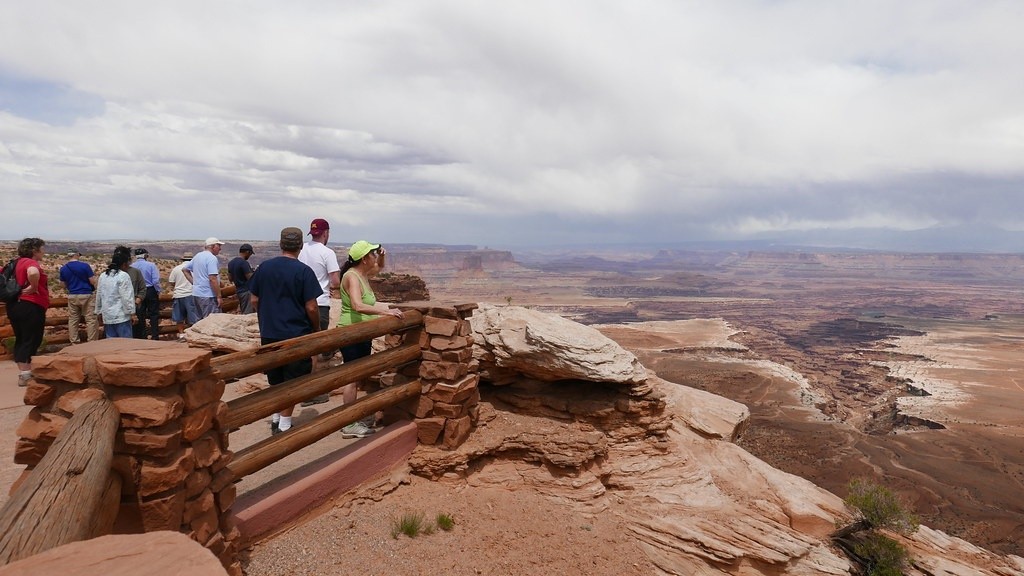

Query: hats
[205,238,226,246]
[180,252,194,260]
[348,240,381,261]
[134,248,149,255]
[281,227,302,244]
[240,244,255,254]
[307,219,330,237]
[67,247,82,256]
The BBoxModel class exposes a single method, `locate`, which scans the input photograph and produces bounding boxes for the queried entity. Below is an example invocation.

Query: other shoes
[271,421,279,431]
[17,373,32,385]
[301,394,329,406]
[272,425,294,435]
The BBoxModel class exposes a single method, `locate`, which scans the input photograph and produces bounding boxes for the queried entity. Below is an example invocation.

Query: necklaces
[299,219,340,406]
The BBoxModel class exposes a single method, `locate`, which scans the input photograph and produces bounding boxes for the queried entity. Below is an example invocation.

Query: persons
[169,252,196,342]
[60,248,99,346]
[95,249,139,339]
[340,242,405,438]
[182,238,225,322]
[247,227,323,435]
[5,238,49,386]
[114,246,147,338]
[130,249,160,339]
[228,244,255,314]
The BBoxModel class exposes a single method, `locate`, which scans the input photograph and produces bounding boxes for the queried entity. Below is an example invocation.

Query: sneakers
[359,415,376,427]
[342,422,376,439]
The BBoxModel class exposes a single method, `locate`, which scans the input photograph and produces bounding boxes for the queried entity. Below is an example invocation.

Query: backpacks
[0,259,30,303]
